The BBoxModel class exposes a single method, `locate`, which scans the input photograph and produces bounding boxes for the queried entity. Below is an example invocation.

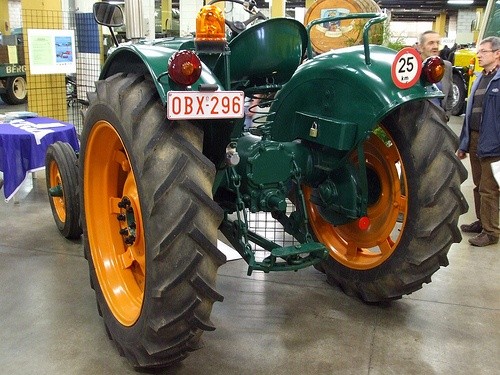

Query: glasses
[476,49,498,53]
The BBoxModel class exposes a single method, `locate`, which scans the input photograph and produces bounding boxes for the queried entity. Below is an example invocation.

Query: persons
[418,29,455,116]
[451,34,500,248]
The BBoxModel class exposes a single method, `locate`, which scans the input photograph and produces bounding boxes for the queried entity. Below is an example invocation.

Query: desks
[0,111,81,205]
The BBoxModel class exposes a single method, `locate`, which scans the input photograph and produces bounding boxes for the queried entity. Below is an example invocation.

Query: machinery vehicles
[0,26,77,107]
[439,1,500,116]
[41,1,473,373]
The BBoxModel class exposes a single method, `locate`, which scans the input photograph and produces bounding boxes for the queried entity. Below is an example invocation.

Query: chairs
[213,17,309,94]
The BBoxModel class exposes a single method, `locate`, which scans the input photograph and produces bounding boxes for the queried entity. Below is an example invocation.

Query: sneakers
[468,233,500,247]
[461,219,482,233]
